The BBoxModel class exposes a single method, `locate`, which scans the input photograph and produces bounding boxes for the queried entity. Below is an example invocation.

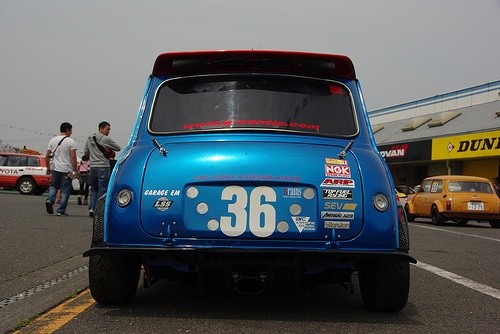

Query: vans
[0,152,52,196]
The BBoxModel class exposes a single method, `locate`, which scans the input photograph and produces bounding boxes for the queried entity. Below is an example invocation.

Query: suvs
[45,158,86,194]
[82,51,418,314]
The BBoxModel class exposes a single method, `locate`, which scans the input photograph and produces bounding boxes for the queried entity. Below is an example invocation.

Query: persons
[109,151,118,175]
[77,160,91,205]
[491,178,500,199]
[84,121,121,216]
[45,122,78,216]
[56,188,62,204]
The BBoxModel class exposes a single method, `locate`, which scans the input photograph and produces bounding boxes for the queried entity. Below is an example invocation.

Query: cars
[396,185,416,214]
[405,175,500,229]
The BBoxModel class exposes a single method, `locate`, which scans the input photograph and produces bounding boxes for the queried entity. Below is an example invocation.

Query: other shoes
[89,209,94,217]
[84,200,88,205]
[45,200,54,214]
[57,211,69,216]
[78,195,82,206]
